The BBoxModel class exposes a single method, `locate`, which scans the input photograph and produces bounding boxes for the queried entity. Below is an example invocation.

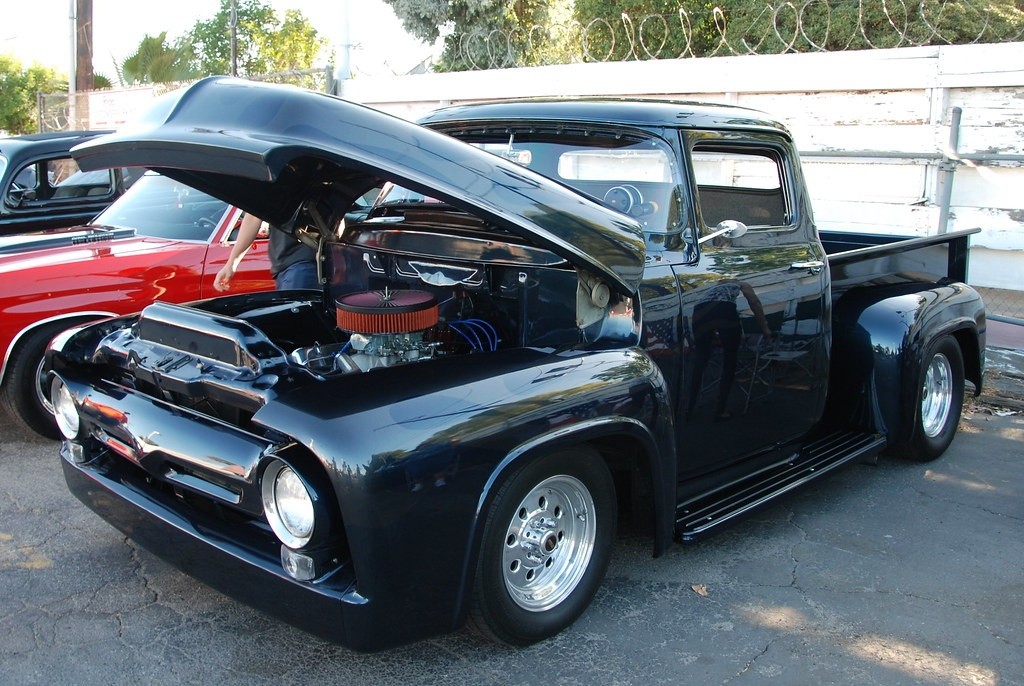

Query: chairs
[52,185,84,198]
[88,187,108,195]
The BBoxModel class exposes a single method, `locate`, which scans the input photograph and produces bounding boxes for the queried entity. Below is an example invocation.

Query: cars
[0,129,243,243]
[0,166,390,446]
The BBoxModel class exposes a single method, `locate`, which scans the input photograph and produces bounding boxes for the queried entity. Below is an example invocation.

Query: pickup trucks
[36,72,988,644]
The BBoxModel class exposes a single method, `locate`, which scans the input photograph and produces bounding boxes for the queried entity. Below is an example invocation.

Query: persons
[213,213,347,290]
[689,278,772,419]
[404,438,461,492]
